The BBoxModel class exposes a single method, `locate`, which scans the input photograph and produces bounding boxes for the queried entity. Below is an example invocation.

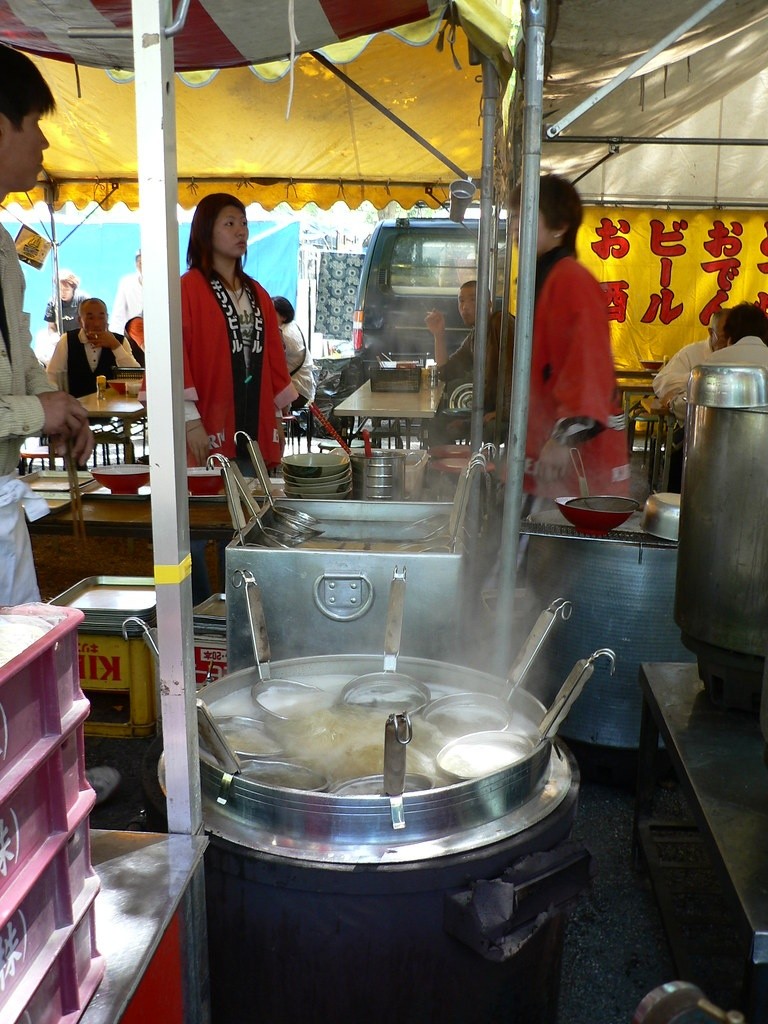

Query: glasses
[712,332,725,344]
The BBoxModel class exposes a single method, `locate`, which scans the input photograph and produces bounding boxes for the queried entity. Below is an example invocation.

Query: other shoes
[82,764,122,805]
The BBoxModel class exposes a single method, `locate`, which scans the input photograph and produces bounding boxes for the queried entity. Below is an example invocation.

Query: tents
[0,0,768,1024]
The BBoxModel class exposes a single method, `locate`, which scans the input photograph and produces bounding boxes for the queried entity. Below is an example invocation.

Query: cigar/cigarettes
[95,334,98,339]
[426,312,432,314]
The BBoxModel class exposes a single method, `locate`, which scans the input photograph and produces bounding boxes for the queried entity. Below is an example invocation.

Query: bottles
[97,374,107,400]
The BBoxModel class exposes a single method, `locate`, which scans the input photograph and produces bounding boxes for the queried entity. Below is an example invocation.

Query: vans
[352,218,509,362]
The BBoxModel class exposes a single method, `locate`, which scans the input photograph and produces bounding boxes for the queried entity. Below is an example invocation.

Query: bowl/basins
[88,465,150,494]
[281,453,353,499]
[639,360,663,371]
[640,493,680,540]
[553,496,636,532]
[185,467,226,495]
[682,363,767,409]
[108,379,141,394]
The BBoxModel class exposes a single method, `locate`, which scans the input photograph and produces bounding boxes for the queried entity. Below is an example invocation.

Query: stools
[318,439,365,453]
[281,407,313,455]
[20,446,66,476]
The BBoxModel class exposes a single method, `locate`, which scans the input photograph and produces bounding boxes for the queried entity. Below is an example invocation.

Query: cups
[125,383,141,399]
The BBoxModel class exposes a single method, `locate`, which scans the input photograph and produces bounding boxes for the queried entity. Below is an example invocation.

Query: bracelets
[187,423,202,433]
[666,397,674,413]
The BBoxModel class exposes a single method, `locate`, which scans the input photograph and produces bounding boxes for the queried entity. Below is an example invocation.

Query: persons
[44,269,88,338]
[108,249,142,337]
[272,296,316,418]
[492,175,634,508]
[45,298,142,471]
[424,281,516,444]
[0,44,94,608]
[181,194,293,477]
[660,300,768,422]
[124,311,145,368]
[651,309,727,492]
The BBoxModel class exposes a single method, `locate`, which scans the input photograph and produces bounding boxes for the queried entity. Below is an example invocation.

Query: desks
[615,371,685,494]
[334,379,445,450]
[77,388,148,469]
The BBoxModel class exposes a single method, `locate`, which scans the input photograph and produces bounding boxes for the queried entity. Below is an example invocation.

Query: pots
[350,452,406,501]
[156,650,571,863]
[674,398,768,658]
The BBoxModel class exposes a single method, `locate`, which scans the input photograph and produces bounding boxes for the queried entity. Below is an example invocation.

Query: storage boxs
[0,606,107,1023]
[72,632,159,739]
